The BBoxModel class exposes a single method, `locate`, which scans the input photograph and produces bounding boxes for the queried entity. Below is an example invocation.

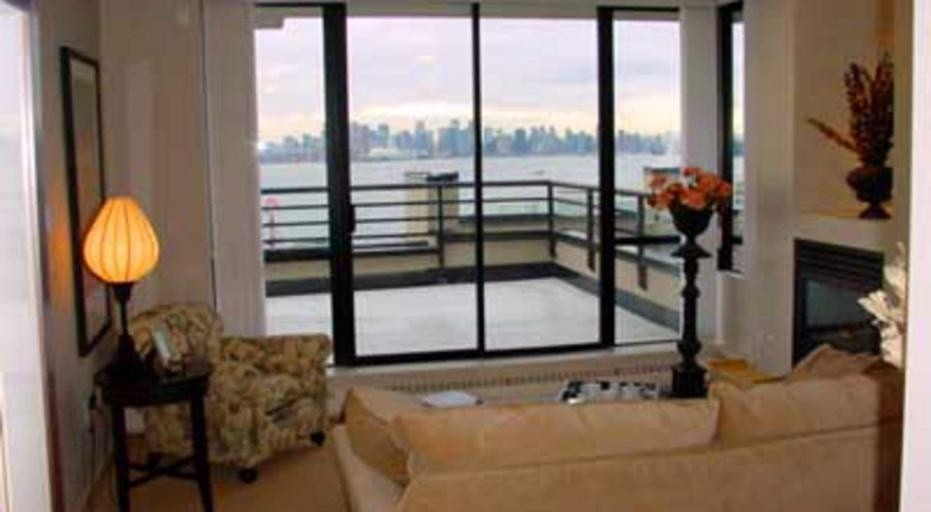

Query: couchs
[329,343,906,511]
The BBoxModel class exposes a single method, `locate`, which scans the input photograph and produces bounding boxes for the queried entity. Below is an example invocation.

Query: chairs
[127,297,334,485]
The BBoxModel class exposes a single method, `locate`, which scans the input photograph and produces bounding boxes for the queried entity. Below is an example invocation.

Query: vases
[668,205,714,257]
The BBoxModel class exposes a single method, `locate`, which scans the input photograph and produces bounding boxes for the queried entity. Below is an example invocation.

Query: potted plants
[805,52,894,220]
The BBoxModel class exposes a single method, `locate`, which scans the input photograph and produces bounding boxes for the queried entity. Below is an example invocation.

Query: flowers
[646,165,735,210]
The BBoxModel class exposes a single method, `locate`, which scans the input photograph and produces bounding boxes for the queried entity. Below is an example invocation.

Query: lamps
[84,196,161,371]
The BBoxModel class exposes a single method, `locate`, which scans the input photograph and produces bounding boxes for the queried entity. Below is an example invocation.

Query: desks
[93,357,217,511]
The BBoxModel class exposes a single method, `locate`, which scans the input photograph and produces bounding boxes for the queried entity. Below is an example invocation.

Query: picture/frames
[59,43,116,358]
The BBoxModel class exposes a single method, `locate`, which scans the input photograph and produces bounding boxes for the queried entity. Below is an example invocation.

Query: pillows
[347,383,437,488]
